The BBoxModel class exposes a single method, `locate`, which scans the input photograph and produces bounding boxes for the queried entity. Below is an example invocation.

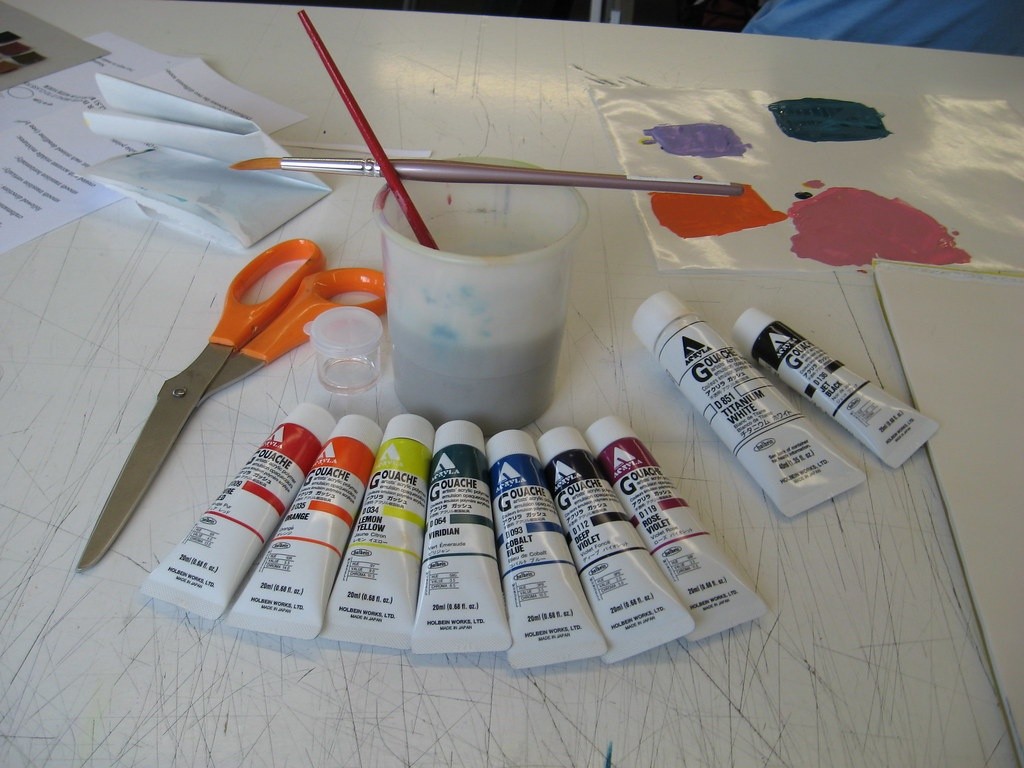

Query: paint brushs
[227,155,747,199]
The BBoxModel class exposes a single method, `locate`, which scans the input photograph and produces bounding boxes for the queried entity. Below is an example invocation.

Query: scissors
[71,238,385,575]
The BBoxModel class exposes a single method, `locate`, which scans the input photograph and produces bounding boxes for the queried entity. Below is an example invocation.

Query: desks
[0,0,1024,768]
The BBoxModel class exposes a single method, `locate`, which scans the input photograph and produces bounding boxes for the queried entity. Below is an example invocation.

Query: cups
[373,156,593,439]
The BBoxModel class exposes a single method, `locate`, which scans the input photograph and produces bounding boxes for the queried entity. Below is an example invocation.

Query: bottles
[304,306,384,395]
